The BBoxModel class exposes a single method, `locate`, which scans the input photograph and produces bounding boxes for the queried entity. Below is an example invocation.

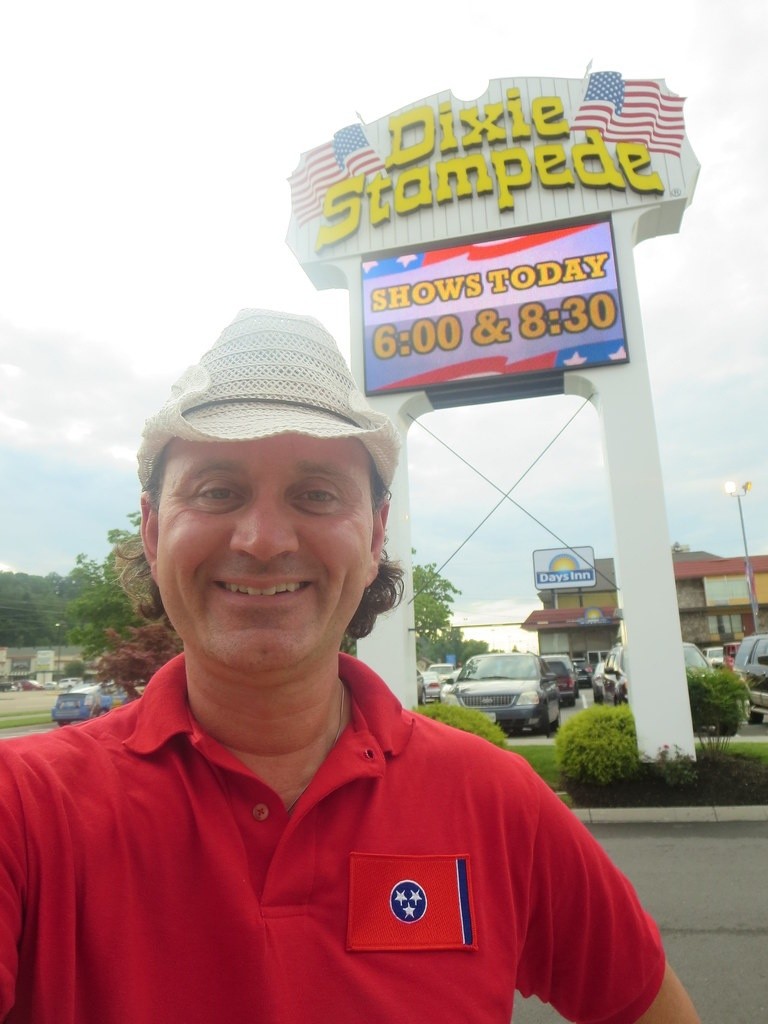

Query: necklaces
[287,680,344,810]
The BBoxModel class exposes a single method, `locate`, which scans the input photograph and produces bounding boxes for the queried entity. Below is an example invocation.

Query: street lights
[56,624,64,680]
[723,480,764,633]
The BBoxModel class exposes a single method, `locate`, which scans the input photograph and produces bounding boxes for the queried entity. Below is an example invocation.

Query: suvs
[734,632,768,724]
[604,643,739,736]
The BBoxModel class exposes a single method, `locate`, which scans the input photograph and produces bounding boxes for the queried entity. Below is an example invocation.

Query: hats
[136,309,403,493]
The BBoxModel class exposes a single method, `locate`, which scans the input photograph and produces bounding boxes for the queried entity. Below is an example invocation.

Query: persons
[0,313,696,1024]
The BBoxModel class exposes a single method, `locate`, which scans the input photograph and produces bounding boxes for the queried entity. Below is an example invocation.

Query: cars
[573,658,593,688]
[591,661,605,702]
[722,643,739,670]
[700,647,724,672]
[444,653,561,735]
[540,654,580,697]
[417,671,426,705]
[14,680,44,691]
[51,681,130,727]
[543,660,576,705]
[423,673,442,702]
[429,665,455,685]
[58,679,82,688]
[43,682,58,689]
[0,678,21,692]
